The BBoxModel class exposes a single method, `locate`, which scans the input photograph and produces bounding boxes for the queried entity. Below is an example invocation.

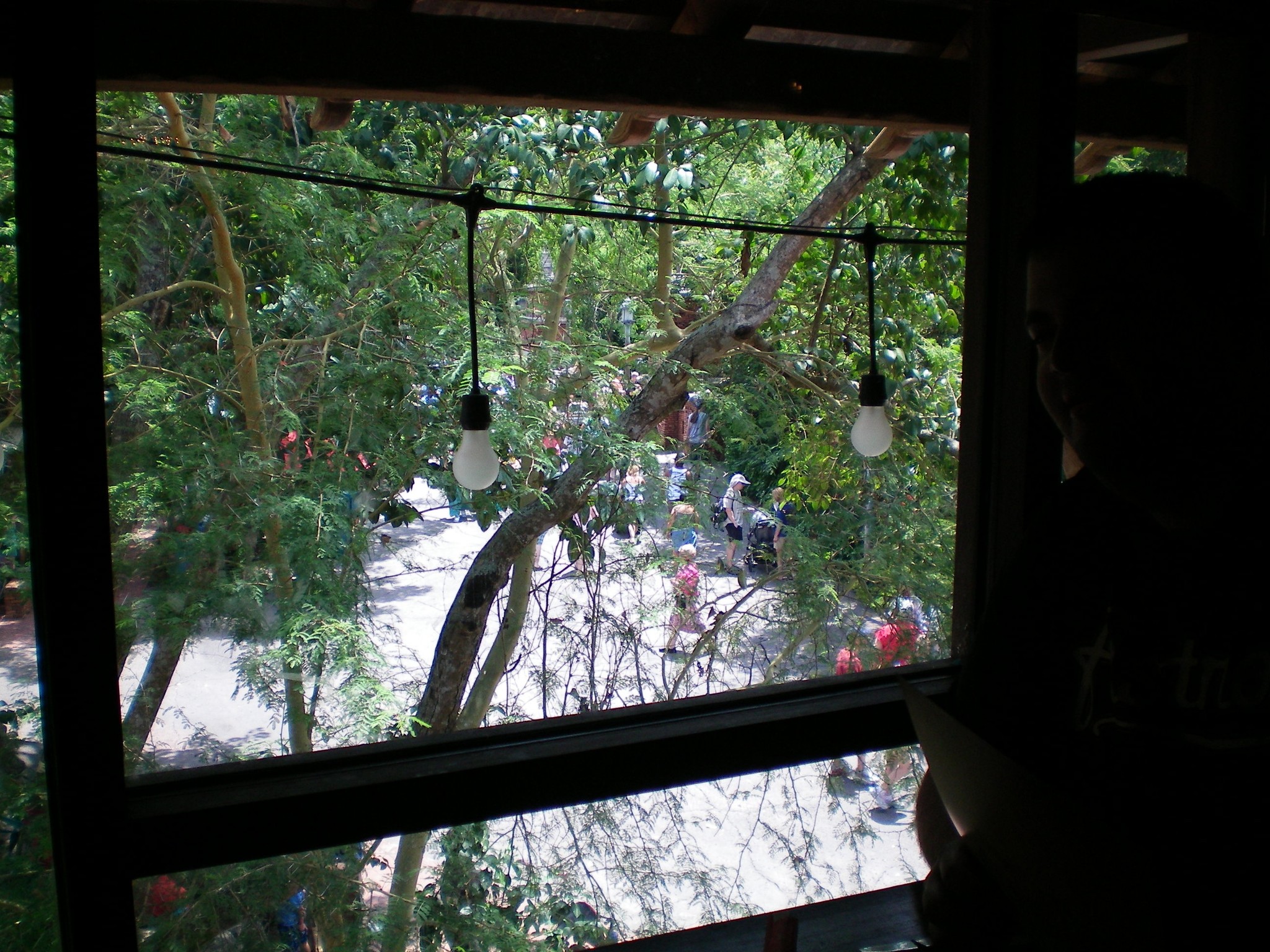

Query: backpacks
[711,494,734,523]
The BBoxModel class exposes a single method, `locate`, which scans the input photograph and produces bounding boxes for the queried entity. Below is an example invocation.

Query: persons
[918,169,1249,936]
[426,379,924,808]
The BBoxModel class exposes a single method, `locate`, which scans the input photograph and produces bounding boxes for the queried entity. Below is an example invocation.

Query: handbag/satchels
[675,595,686,610]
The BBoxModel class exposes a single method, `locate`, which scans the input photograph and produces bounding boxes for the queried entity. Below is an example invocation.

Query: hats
[676,452,685,463]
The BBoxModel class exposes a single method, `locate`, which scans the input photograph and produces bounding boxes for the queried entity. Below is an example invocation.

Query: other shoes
[871,787,905,810]
[853,768,875,784]
[726,564,743,575]
[788,576,793,580]
[829,767,854,777]
[776,577,783,580]
[659,647,677,654]
